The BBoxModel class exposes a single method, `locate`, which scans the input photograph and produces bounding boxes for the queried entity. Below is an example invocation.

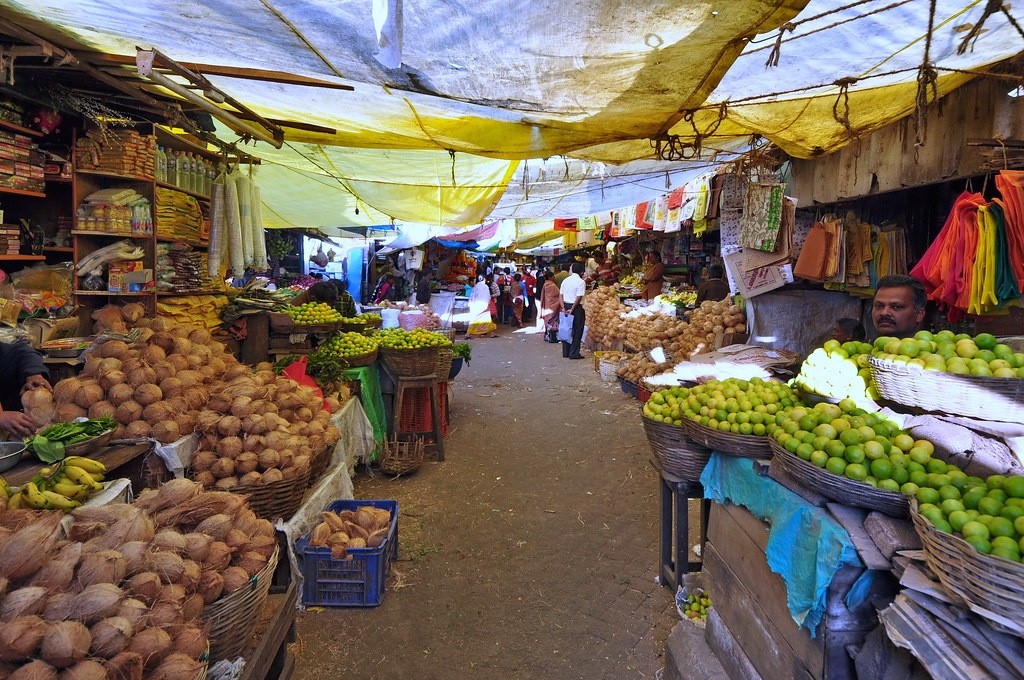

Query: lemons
[684,590,714,623]
[280,301,453,356]
[790,330,1024,401]
[642,377,1024,566]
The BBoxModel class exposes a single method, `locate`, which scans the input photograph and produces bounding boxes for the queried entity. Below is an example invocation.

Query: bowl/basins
[34,347,85,357]
[25,424,118,460]
[0,442,28,474]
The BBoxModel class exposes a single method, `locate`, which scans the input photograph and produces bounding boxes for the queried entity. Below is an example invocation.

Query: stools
[650,459,712,592]
[390,373,444,461]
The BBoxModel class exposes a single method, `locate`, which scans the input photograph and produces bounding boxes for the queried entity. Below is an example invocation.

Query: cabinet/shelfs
[0,118,73,261]
[71,121,231,317]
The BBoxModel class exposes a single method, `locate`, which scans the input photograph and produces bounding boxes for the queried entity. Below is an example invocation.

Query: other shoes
[581,356,585,359]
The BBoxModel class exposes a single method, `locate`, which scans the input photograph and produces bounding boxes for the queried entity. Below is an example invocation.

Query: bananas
[8,456,107,512]
[0,475,12,502]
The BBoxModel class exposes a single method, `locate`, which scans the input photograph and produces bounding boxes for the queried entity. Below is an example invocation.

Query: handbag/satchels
[558,312,574,345]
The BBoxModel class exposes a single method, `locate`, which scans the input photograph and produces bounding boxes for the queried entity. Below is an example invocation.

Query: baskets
[194,543,280,665]
[907,496,1024,639]
[23,418,120,464]
[796,386,886,416]
[297,322,341,334]
[638,401,713,484]
[340,323,367,335]
[307,445,337,487]
[380,341,439,376]
[205,463,312,525]
[598,358,619,383]
[867,354,1024,424]
[192,638,209,680]
[767,432,912,521]
[678,410,774,460]
[366,319,383,329]
[434,348,453,383]
[339,348,380,367]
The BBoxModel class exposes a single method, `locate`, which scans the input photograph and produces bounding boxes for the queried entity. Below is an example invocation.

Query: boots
[548,331,560,343]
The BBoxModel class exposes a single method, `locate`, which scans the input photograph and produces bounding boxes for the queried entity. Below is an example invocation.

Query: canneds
[75,204,152,232]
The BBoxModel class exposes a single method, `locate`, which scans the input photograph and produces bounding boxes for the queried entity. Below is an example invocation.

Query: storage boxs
[294,499,398,607]
[617,376,652,402]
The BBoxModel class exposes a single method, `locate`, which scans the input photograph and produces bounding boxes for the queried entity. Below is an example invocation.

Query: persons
[342,273,350,290]
[638,250,664,300]
[416,266,433,304]
[437,256,450,274]
[291,281,338,305]
[864,273,926,345]
[464,253,621,343]
[309,272,323,280]
[832,318,866,345]
[694,265,731,309]
[559,263,587,360]
[0,339,54,442]
[371,266,398,304]
[328,278,358,318]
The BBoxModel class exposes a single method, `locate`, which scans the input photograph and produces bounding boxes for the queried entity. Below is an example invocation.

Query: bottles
[116,206,124,218]
[86,218,95,231]
[93,204,105,218]
[106,219,117,232]
[176,151,190,192]
[117,220,125,233]
[210,161,216,183]
[155,144,162,181]
[195,154,203,195]
[160,146,168,183]
[124,207,132,220]
[175,150,179,158]
[200,157,206,195]
[187,152,196,193]
[75,218,86,230]
[95,219,105,231]
[204,159,211,196]
[166,148,176,186]
[78,204,95,217]
[124,220,132,233]
[106,206,117,218]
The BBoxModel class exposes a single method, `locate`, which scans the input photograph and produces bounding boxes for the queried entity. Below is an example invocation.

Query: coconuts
[0,301,390,680]
[584,283,747,384]
